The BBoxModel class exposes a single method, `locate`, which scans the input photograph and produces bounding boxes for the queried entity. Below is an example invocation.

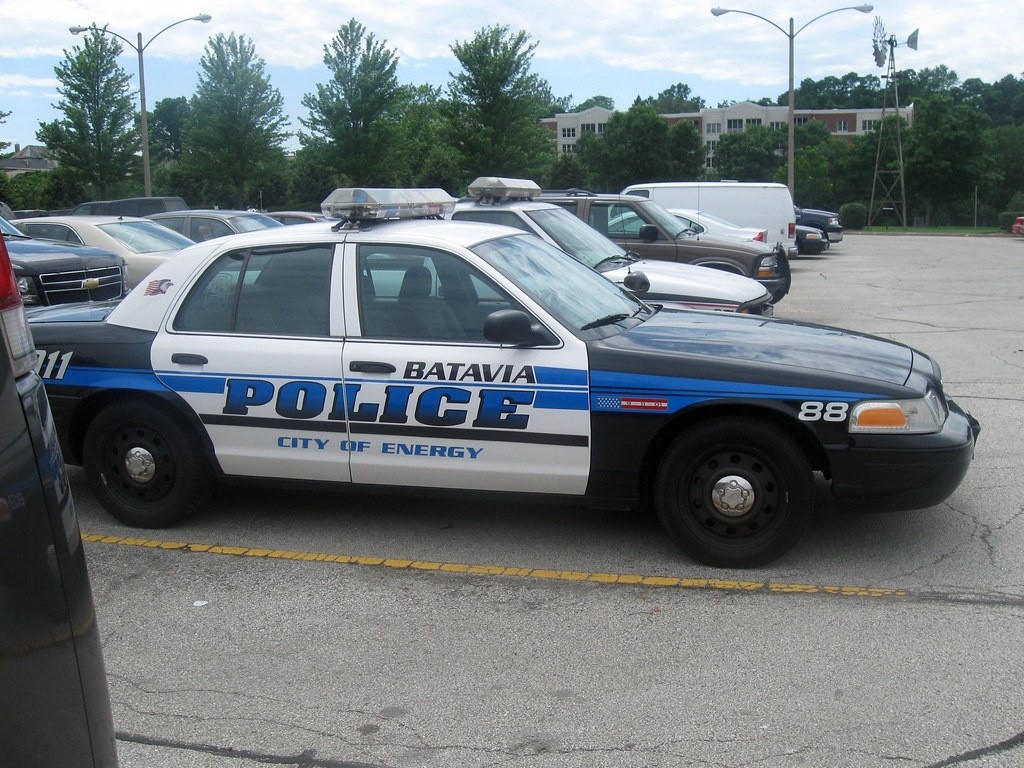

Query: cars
[25,187,982,570]
[793,202,844,243]
[262,211,324,226]
[795,224,830,254]
[142,210,285,244]
[1012,217,1024,234]
[9,215,198,295]
[12,210,49,219]
[0,201,16,220]
[0,231,119,768]
[608,207,768,244]
[0,214,131,305]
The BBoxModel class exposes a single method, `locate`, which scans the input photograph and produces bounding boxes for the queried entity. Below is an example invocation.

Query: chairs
[360,277,400,338]
[396,267,465,341]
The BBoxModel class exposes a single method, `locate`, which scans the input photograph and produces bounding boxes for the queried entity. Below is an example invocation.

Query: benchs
[222,288,329,336]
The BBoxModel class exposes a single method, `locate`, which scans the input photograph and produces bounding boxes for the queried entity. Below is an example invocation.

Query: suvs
[457,189,791,303]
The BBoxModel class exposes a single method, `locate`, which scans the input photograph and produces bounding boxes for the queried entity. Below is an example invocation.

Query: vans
[69,197,190,218]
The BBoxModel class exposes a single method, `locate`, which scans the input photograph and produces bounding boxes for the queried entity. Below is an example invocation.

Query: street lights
[711,3,874,202]
[69,15,212,197]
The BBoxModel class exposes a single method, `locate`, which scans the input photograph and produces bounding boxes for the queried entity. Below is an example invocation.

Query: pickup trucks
[211,176,774,322]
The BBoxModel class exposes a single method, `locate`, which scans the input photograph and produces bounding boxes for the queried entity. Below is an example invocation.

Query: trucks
[608,183,799,260]
